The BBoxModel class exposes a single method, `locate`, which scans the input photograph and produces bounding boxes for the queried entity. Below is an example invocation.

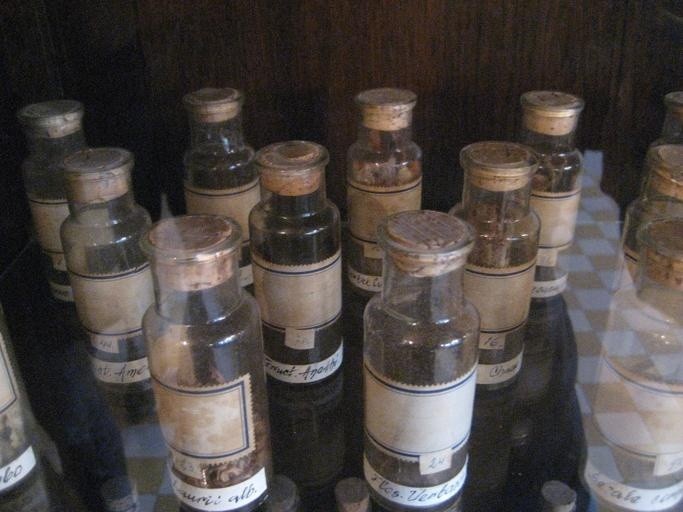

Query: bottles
[56,270,59,270]
[139,213,279,510]
[345,89,425,293]
[448,142,541,389]
[19,101,89,305]
[182,88,262,291]
[362,211,482,511]
[249,140,345,387]
[584,218,683,511]
[592,141,682,458]
[514,92,581,301]
[56,148,156,393]
